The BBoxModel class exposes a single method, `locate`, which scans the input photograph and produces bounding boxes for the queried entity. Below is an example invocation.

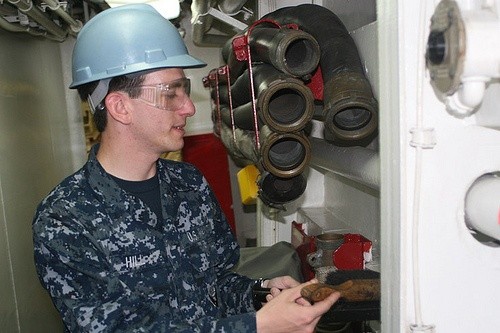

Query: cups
[317,234,344,266]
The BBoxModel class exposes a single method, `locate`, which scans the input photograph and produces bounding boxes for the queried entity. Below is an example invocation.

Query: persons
[32,3,340,333]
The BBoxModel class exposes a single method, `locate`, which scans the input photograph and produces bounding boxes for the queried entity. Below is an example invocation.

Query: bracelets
[252,276,270,312]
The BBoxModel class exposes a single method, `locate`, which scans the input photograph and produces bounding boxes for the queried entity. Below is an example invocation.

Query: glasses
[121,78,191,111]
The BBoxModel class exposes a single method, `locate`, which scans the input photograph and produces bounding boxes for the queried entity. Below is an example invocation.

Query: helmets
[68,3,208,89]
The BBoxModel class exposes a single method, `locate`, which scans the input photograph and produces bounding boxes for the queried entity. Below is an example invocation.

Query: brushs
[301,269,381,301]
